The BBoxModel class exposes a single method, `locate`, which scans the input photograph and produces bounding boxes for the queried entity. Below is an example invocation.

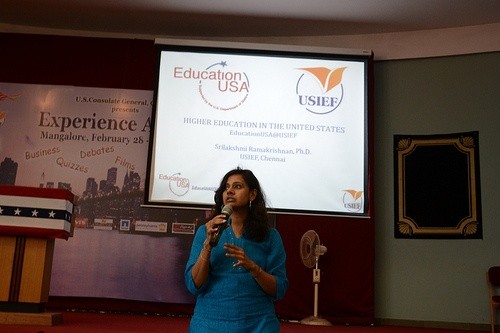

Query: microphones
[209,204,233,247]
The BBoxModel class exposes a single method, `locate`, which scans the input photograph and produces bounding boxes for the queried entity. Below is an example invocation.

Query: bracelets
[253,265,262,277]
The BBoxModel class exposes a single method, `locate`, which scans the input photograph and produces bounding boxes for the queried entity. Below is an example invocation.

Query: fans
[297,230,333,326]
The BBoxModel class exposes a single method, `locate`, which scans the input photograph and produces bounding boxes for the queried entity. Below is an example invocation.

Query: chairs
[487,266,500,333]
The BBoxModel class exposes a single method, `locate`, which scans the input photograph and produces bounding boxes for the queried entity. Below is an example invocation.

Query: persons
[183,166,289,333]
